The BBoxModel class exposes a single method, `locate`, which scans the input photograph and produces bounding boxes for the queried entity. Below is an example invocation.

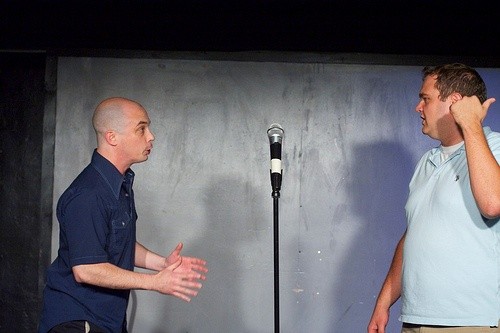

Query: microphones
[267,123,285,191]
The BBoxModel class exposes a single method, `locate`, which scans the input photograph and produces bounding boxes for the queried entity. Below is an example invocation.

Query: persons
[367,61,500,333]
[40,97,208,333]
